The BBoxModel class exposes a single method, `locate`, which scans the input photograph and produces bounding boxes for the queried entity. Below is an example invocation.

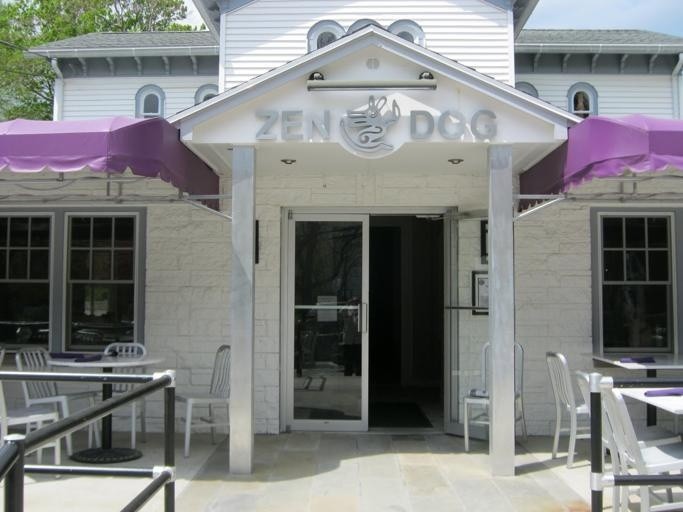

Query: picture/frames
[469,270,490,316]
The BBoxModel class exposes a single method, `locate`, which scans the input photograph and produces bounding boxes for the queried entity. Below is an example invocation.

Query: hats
[348,297,361,303]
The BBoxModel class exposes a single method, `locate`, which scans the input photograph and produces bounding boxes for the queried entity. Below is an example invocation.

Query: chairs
[462,337,526,451]
[0,343,231,478]
[545,350,683,510]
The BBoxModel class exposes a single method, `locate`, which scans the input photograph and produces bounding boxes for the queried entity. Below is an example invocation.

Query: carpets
[310,399,433,429]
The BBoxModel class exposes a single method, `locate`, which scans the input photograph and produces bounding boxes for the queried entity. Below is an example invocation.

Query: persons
[338,297,362,377]
